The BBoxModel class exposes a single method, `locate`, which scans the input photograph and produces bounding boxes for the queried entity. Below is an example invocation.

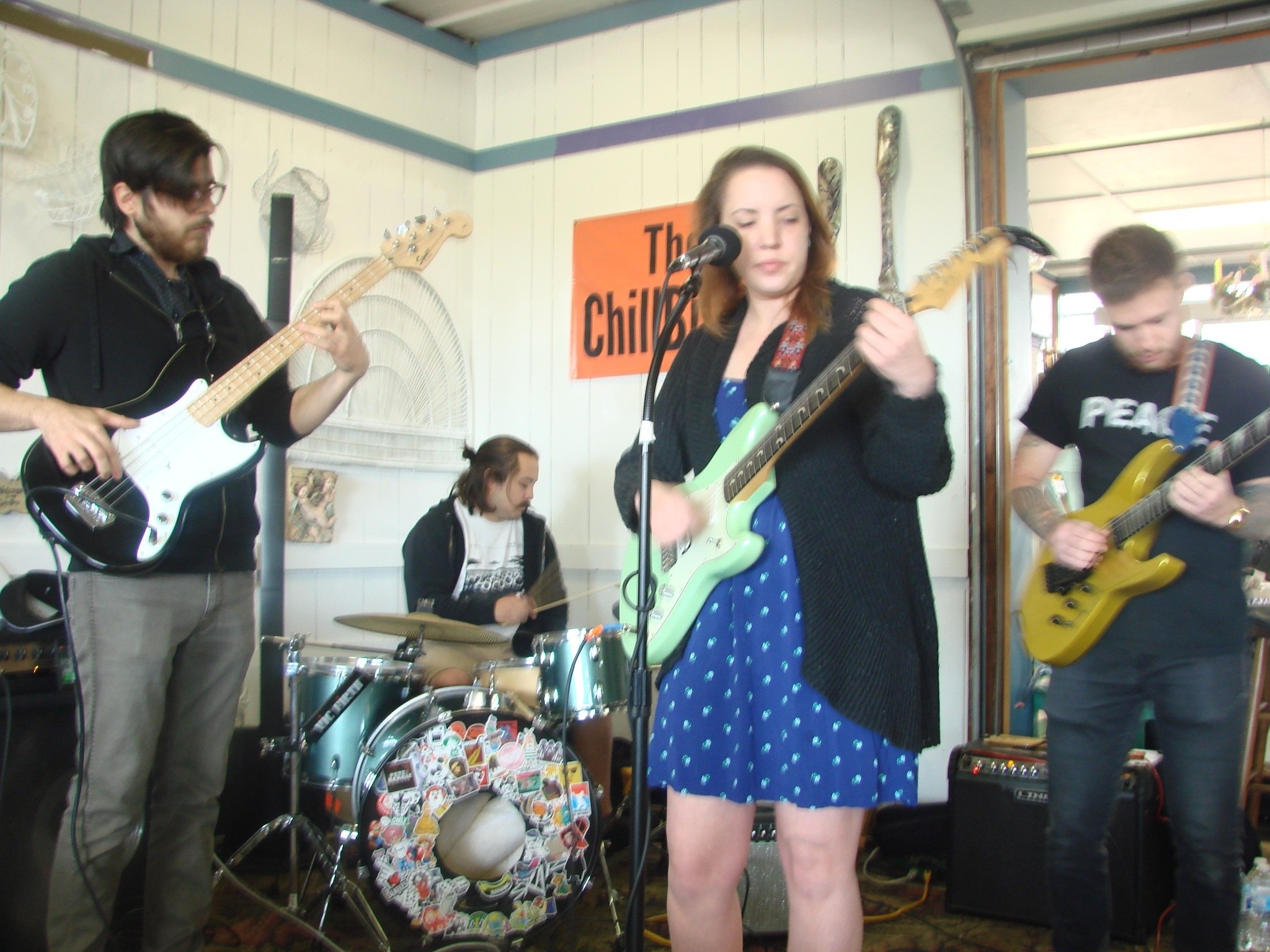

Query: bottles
[1236,856,1270,952]
[1034,669,1050,736]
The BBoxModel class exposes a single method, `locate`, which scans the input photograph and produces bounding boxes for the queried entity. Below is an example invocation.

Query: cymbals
[331,609,511,647]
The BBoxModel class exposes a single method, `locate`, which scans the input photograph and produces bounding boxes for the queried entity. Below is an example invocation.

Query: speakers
[948,736,1172,944]
[736,803,788,940]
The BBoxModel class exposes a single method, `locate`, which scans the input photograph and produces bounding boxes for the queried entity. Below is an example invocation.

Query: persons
[613,146,953,952]
[401,435,631,843]
[0,108,367,952]
[1008,222,1270,952]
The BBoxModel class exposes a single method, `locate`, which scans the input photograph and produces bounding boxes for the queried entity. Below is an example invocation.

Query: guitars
[1019,408,1269,667]
[16,208,475,577]
[617,222,1012,669]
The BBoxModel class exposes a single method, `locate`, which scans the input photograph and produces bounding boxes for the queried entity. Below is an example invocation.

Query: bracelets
[1218,501,1249,534]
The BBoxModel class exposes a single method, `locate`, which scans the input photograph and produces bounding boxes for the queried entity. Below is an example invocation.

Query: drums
[353,684,606,952]
[532,620,633,722]
[477,655,617,822]
[257,632,425,794]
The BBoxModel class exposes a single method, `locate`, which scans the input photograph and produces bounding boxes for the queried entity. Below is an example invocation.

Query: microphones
[668,226,741,272]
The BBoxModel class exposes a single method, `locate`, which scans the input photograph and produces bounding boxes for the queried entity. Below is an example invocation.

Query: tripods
[208,626,427,952]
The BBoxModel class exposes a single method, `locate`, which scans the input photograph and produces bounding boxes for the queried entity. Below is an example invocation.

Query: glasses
[144,181,226,213]
